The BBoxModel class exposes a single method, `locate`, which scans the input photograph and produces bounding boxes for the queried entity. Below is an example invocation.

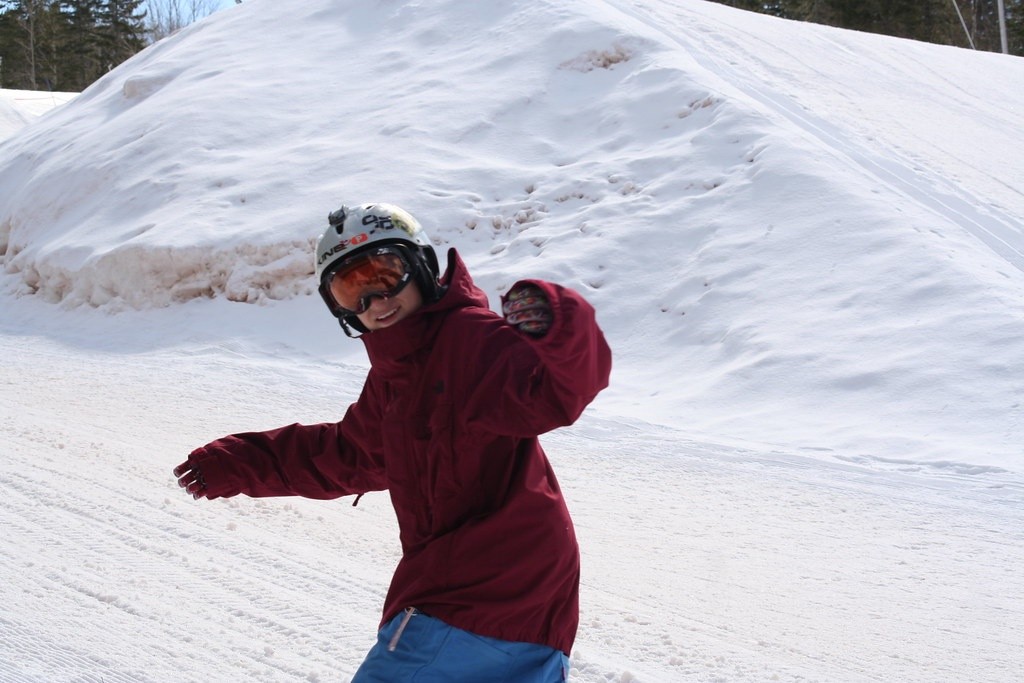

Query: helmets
[314,203,439,335]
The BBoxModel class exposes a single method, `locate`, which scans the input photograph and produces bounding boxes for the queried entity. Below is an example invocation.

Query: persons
[173,203,611,683]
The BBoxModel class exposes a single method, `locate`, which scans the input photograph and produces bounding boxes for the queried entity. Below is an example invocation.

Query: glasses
[323,242,417,316]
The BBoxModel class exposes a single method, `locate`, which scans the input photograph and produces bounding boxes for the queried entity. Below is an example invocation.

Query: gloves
[503,285,552,334]
[173,458,209,500]
